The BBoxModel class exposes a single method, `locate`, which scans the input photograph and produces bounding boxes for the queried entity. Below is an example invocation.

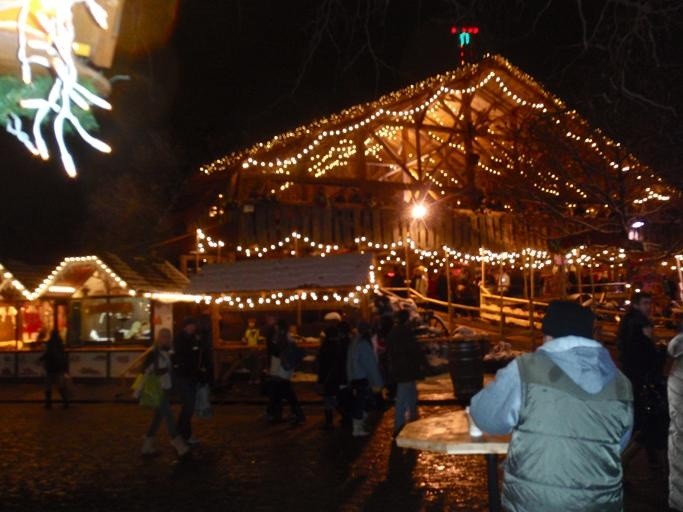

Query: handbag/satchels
[136,372,165,410]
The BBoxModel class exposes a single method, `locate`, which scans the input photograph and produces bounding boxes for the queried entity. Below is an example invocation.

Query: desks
[396,410,511,512]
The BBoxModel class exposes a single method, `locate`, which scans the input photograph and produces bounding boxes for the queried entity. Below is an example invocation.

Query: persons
[262,319,308,426]
[664,311,682,511]
[618,316,668,473]
[309,291,438,448]
[467,296,636,511]
[238,316,262,346]
[36,329,70,411]
[167,315,207,445]
[610,289,659,432]
[134,327,194,457]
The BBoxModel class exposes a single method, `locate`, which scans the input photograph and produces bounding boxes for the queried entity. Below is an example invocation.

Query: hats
[540,300,592,336]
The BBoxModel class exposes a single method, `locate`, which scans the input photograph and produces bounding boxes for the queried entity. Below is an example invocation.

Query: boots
[140,430,202,459]
[325,410,377,440]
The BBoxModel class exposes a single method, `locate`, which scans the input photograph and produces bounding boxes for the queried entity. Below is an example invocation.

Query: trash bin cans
[447,340,486,401]
